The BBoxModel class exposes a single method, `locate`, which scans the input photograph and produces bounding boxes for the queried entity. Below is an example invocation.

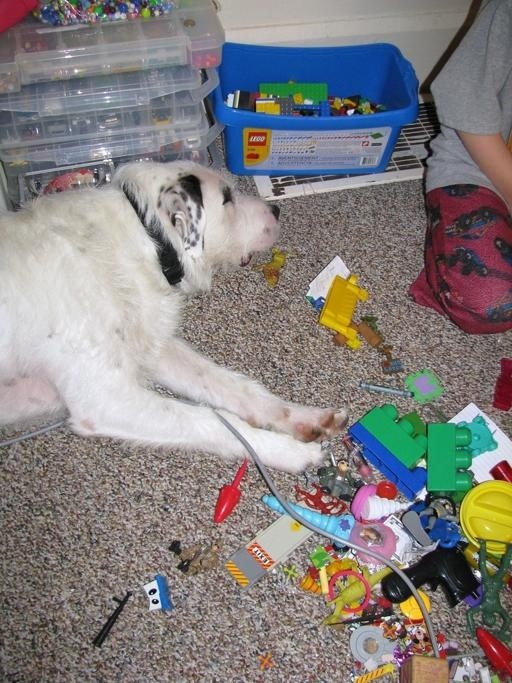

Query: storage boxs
[210,41,418,175]
[2,3,226,209]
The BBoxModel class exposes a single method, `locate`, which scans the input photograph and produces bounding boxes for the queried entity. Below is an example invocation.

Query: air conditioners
[0,155,349,477]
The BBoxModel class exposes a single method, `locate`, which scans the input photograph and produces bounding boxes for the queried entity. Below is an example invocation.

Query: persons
[317,459,353,502]
[407,0,512,333]
